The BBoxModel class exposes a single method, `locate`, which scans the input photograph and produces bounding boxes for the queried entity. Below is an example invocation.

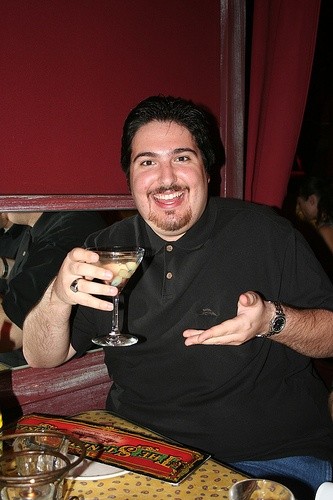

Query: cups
[229,478,295,500]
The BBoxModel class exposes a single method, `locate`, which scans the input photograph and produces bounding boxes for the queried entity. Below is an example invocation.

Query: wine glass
[86,244,146,347]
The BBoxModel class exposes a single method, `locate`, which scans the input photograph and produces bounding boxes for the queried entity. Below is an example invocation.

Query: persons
[0,211,116,352]
[294,174,333,283]
[20,94,333,500]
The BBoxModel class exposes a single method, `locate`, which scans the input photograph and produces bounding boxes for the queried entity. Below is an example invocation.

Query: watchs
[257,301,286,338]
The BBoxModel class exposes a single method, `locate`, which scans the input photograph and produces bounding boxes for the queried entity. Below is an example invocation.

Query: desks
[0,410,252,500]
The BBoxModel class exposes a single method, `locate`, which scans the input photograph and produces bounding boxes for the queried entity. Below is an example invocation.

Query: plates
[64,454,129,481]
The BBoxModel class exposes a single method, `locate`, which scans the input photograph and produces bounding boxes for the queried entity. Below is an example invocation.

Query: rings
[70,278,83,293]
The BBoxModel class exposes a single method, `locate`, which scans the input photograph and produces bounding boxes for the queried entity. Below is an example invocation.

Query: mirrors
[0,209,139,371]
